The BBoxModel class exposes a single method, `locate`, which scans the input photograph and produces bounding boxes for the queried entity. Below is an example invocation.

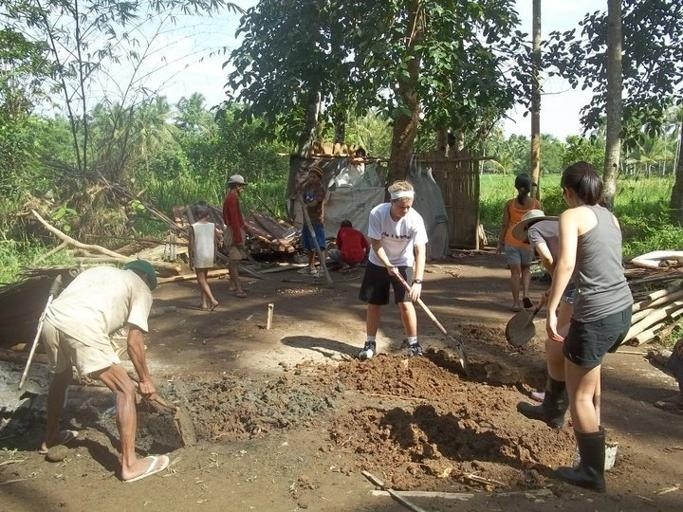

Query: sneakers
[512,297,533,313]
[529,391,545,401]
[409,343,422,357]
[358,342,376,358]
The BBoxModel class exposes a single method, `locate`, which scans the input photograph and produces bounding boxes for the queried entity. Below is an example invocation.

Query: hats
[511,209,560,240]
[227,176,249,186]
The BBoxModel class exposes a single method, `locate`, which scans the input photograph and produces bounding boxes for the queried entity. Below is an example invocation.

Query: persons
[298,166,329,276]
[39,260,170,482]
[327,218,368,270]
[357,180,428,359]
[221,174,248,298]
[512,208,577,400]
[495,173,544,312]
[516,160,635,491]
[188,201,222,312]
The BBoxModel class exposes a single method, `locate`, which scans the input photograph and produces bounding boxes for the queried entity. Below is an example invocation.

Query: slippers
[39,430,78,453]
[122,454,169,482]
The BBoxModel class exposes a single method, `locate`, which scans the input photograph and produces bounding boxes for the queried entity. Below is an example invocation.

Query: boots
[557,427,608,492]
[518,376,569,429]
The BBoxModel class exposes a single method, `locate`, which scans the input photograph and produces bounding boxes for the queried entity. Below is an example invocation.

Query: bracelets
[413,276,422,285]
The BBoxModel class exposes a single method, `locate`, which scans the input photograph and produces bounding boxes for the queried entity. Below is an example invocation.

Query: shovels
[391,268,468,376]
[505,287,550,346]
[131,379,185,445]
